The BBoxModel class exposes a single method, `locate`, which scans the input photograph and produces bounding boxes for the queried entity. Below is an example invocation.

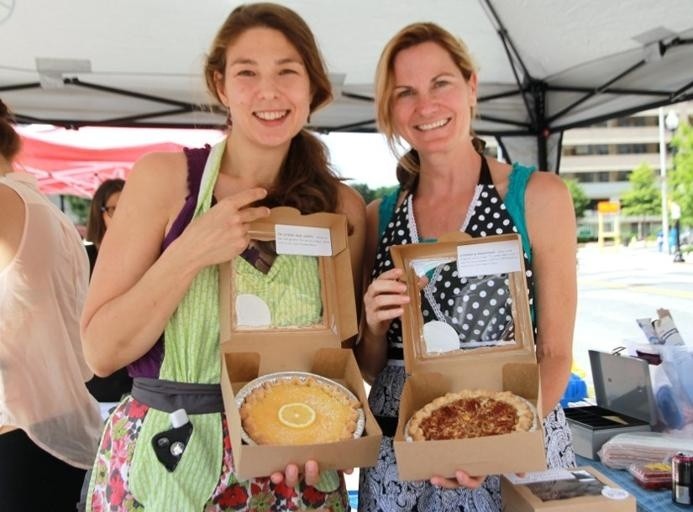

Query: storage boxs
[389,231,552,483]
[218,211,383,478]
[557,347,662,461]
[500,465,637,511]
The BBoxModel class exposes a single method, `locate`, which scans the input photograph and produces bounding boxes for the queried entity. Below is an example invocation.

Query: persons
[83,3,370,512]
[354,19,583,510]
[81,174,134,402]
[0,97,108,512]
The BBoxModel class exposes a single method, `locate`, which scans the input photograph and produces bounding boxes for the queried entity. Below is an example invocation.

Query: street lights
[660,109,681,252]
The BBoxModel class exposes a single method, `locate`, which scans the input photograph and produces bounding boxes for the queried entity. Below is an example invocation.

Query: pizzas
[407,389,534,441]
[238,377,362,446]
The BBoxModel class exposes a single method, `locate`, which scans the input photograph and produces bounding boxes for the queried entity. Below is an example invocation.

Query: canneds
[671,451,693,507]
